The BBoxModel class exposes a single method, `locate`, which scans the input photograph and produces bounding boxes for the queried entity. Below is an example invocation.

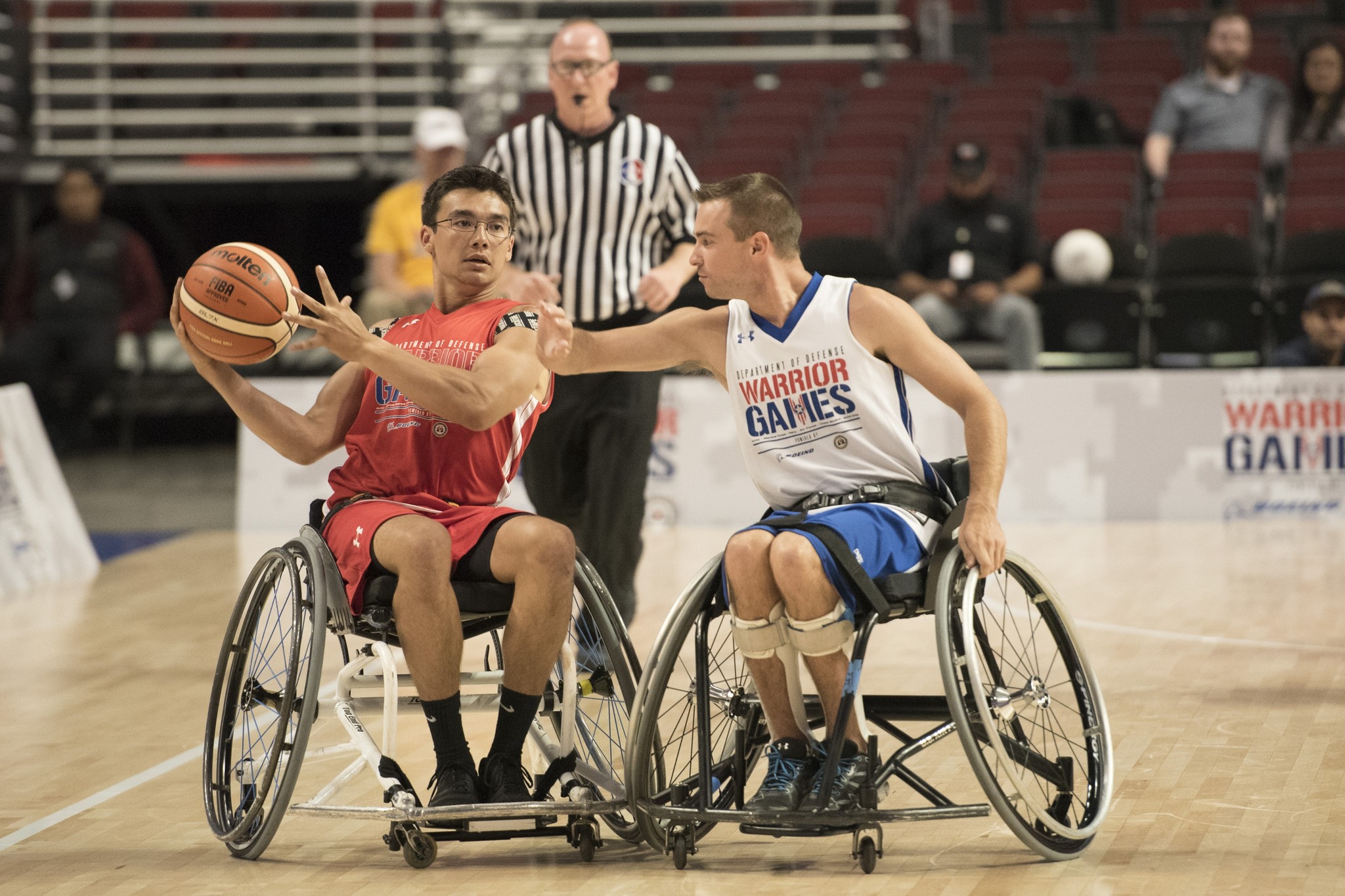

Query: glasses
[553,59,616,79]
[435,216,517,239]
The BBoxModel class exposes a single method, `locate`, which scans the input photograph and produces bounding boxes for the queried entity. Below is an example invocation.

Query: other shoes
[577,638,608,673]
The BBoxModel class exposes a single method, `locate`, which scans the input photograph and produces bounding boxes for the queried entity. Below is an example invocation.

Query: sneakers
[479,755,534,805]
[426,756,480,832]
[799,737,891,832]
[742,736,827,825]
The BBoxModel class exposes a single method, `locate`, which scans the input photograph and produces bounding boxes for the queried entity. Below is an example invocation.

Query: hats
[1302,279,1345,308]
[951,140,988,182]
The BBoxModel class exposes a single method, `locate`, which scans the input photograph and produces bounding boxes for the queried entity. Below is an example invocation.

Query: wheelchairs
[202,499,666,868]
[626,455,1114,873]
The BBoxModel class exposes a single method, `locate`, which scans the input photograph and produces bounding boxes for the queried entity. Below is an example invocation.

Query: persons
[0,159,163,431]
[888,145,1051,376]
[532,171,1010,821]
[1142,10,1288,226]
[361,104,471,304]
[169,164,576,802]
[1260,275,1345,368]
[477,19,705,694]
[1289,37,1345,155]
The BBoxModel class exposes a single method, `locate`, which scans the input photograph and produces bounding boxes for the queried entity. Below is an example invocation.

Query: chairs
[0,0,1345,436]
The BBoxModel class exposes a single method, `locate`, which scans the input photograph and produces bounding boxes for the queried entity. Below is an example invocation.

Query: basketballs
[179,241,303,365]
